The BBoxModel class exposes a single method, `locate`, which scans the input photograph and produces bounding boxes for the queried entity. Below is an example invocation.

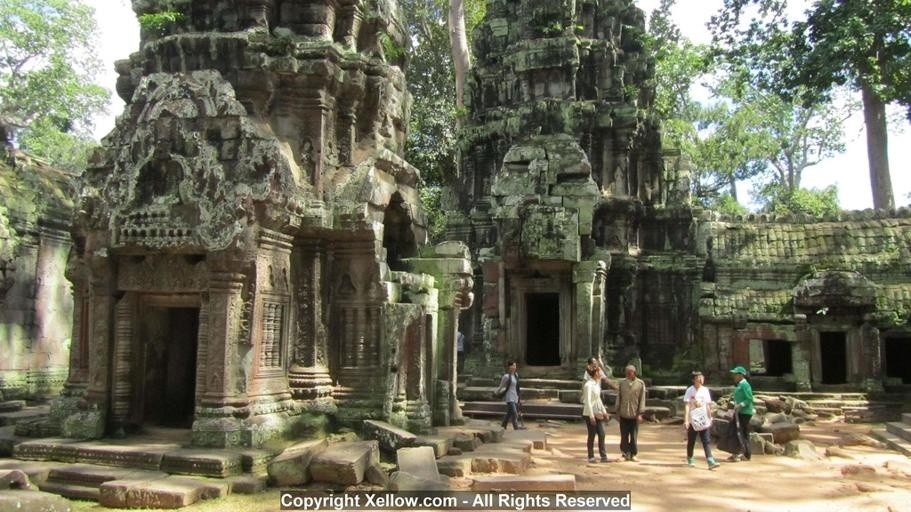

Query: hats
[729,366,747,376]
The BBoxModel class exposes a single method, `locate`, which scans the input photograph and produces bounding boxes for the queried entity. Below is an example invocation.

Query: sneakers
[687,453,751,470]
[588,455,640,464]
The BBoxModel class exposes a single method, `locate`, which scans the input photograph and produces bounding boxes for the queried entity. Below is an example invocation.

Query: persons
[683,369,720,470]
[724,366,753,462]
[493,359,527,430]
[457,328,465,376]
[581,357,646,463]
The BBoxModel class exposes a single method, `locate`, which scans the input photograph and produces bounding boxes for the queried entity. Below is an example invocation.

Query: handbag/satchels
[688,407,712,432]
